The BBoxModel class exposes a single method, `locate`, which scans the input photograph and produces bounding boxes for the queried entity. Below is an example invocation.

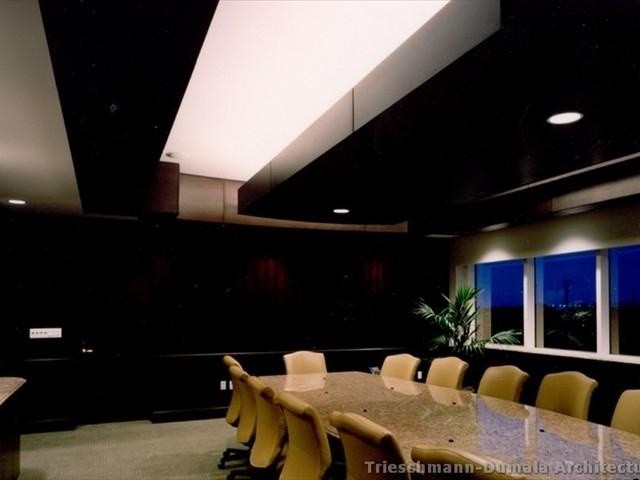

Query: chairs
[218,350,640,480]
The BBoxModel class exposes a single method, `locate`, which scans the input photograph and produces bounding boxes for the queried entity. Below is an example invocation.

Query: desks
[0,376,27,480]
